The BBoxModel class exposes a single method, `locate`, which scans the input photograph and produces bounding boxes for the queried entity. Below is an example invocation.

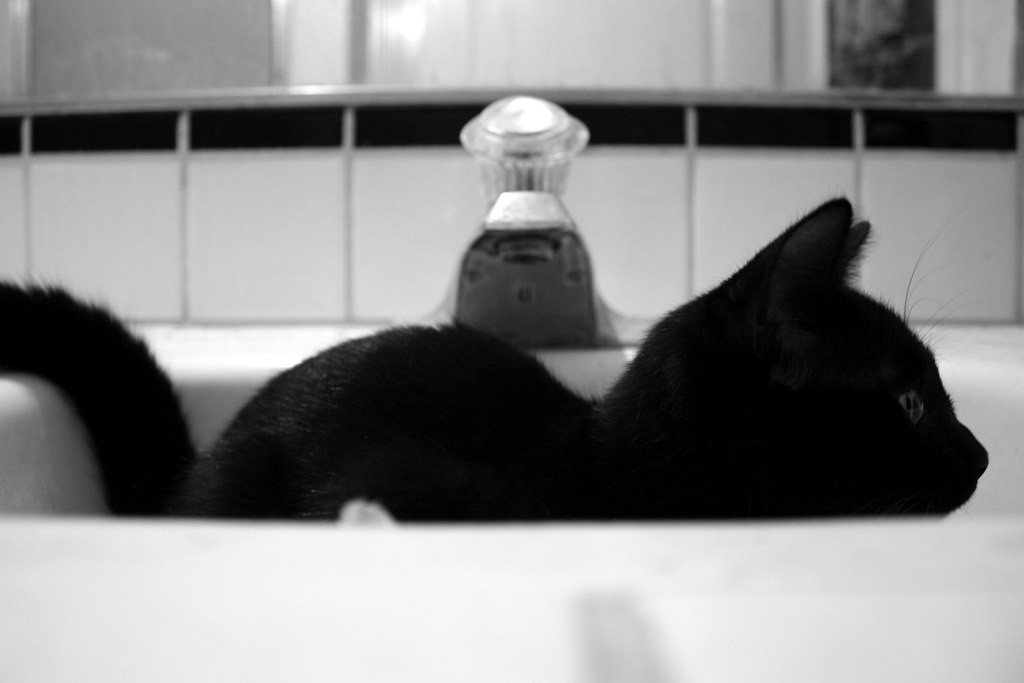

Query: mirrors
[0,0,1024,116]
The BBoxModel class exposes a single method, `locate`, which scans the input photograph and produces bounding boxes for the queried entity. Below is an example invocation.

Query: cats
[1,195,991,524]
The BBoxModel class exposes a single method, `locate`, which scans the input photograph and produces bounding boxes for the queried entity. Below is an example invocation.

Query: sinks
[1,320,1024,681]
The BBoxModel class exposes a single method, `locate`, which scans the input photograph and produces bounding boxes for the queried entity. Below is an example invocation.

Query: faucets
[448,92,597,350]
